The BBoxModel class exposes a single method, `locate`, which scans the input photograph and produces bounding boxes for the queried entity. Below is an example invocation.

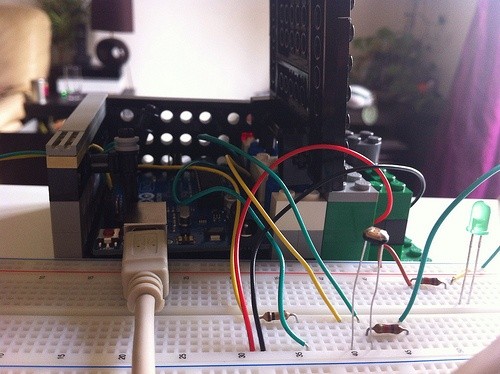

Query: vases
[346,110,441,196]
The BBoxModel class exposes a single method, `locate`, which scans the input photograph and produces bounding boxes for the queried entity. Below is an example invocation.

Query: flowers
[347,24,450,119]
